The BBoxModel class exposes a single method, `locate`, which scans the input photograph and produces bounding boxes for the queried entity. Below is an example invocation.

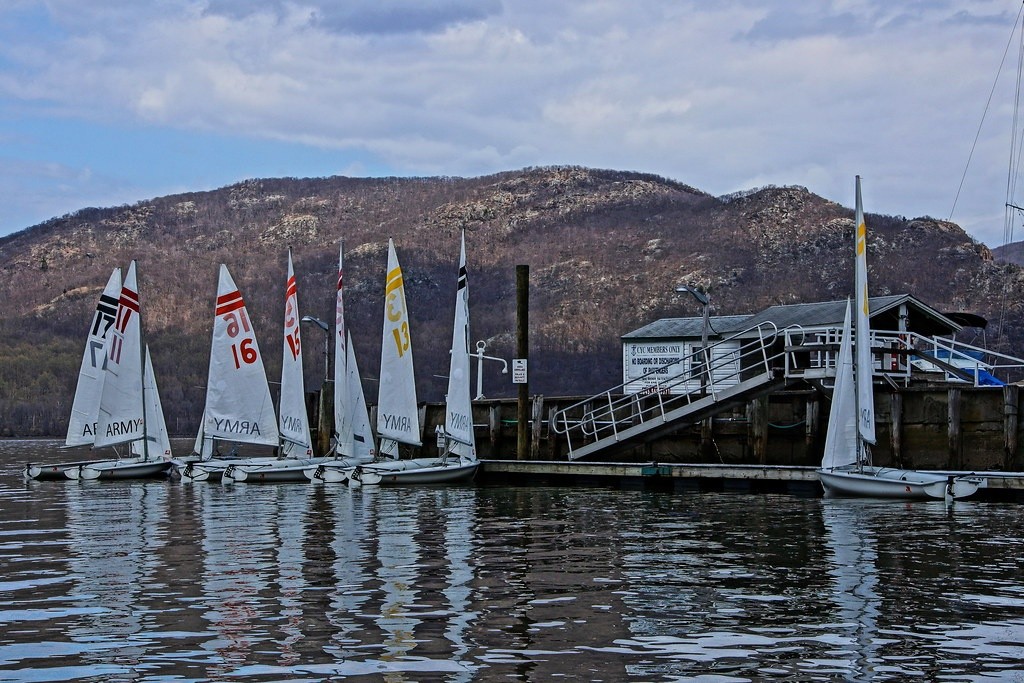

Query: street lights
[675,285,708,462]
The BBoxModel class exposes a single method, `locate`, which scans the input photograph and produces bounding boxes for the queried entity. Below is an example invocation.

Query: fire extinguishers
[891,347,898,371]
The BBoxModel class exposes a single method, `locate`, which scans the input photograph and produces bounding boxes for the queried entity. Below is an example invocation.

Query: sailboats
[170,264,280,466]
[21,267,123,480]
[340,229,480,485]
[813,175,983,499]
[178,245,314,482]
[302,235,423,483]
[221,236,375,484]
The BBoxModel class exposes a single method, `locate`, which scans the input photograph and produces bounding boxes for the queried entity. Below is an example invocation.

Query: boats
[913,311,1004,385]
[62,258,172,481]
